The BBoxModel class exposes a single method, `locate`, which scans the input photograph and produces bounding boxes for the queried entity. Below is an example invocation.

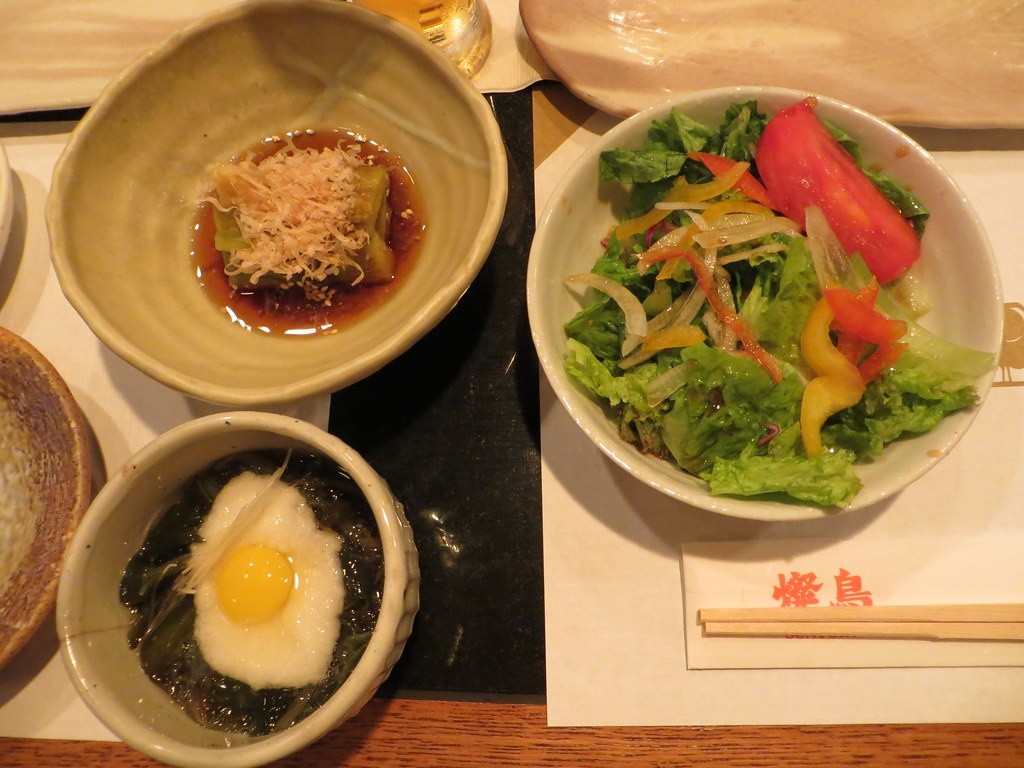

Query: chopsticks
[699,604,1024,641]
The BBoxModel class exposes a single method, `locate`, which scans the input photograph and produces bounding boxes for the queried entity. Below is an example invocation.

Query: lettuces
[561,98,995,513]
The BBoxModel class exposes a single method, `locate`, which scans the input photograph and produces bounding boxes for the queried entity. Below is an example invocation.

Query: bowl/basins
[53,411,420,767]
[43,0,510,407]
[526,88,1004,522]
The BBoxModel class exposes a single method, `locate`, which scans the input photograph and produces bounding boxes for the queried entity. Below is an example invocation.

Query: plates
[519,0,1023,129]
[0,326,93,667]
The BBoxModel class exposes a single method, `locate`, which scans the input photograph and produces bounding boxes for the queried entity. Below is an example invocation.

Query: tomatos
[756,98,918,282]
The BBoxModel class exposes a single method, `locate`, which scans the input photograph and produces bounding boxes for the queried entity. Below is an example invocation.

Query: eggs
[188,470,346,688]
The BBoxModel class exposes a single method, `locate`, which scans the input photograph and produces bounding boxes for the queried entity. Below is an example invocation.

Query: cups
[347,0,491,80]
